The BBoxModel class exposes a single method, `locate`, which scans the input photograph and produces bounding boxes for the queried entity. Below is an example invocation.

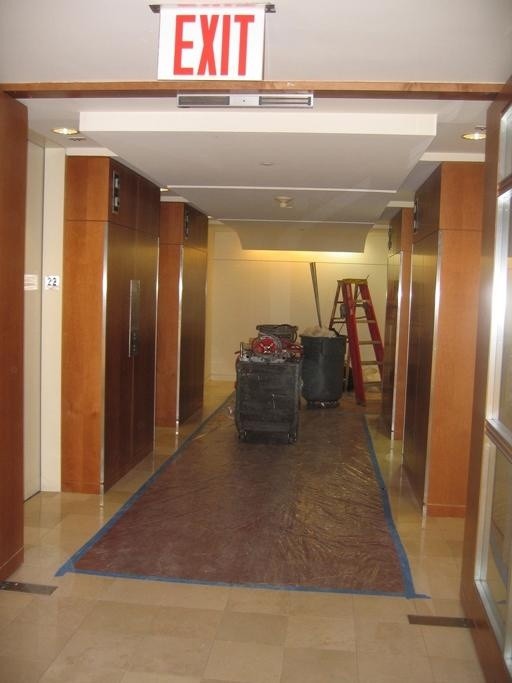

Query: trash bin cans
[300,333,346,409]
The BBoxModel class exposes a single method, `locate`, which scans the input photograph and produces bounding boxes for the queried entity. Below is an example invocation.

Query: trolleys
[231,322,305,448]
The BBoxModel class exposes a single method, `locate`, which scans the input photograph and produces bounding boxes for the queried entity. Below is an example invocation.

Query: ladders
[329,278,384,403]
